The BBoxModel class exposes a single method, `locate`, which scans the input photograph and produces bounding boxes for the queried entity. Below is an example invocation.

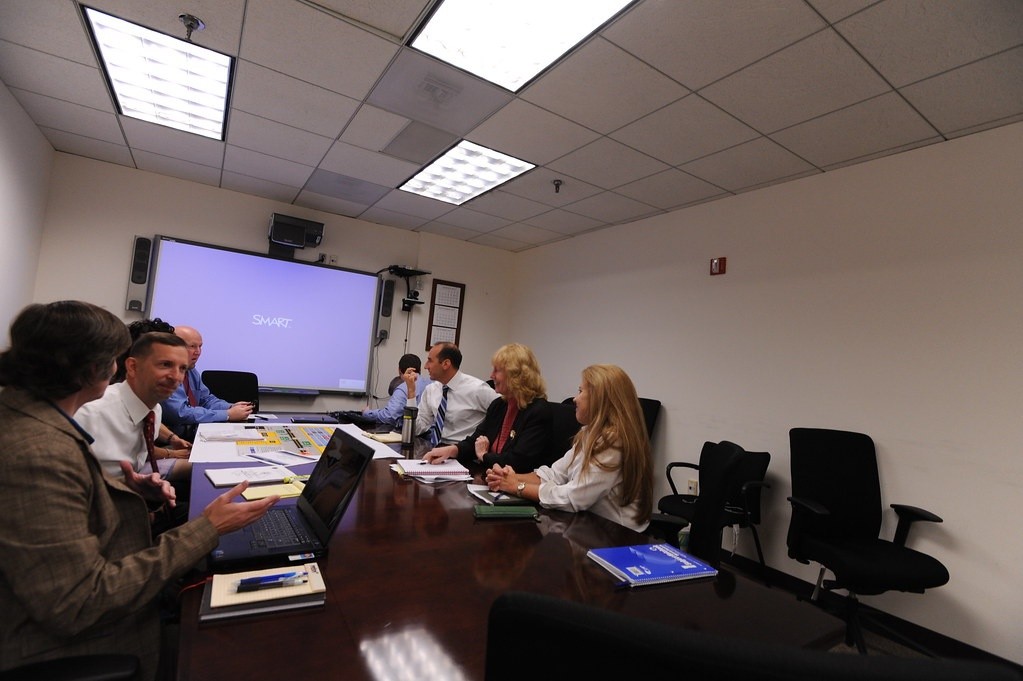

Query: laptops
[211,428,375,569]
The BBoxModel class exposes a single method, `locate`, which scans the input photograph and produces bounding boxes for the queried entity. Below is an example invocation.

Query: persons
[362,354,430,431]
[0,301,283,680]
[422,343,554,472]
[161,326,253,429]
[114,316,191,479]
[72,330,188,527]
[403,342,501,448]
[487,363,648,536]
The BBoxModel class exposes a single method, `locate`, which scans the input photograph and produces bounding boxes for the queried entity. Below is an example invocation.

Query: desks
[173,411,979,681]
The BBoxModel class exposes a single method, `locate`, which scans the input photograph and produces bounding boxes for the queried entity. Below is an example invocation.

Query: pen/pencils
[255,415,268,421]
[419,460,448,465]
[322,416,325,420]
[494,491,505,501]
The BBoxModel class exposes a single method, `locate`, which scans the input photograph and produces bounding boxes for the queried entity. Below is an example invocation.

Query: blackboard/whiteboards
[147,234,382,395]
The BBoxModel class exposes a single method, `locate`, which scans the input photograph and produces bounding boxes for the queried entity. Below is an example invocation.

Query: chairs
[785,427,950,655]
[559,398,662,497]
[201,370,259,417]
[658,440,771,582]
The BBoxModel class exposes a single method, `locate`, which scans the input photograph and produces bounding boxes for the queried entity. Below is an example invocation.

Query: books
[362,431,402,444]
[587,543,717,587]
[204,465,298,489]
[397,459,469,475]
[240,481,305,501]
[198,563,327,623]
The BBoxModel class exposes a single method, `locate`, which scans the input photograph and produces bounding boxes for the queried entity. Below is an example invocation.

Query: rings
[409,374,410,376]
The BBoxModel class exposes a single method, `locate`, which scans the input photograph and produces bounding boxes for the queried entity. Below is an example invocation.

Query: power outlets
[687,479,697,495]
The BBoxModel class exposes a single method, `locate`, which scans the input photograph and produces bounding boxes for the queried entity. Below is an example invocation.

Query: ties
[183,370,197,407]
[143,411,159,473]
[429,384,449,447]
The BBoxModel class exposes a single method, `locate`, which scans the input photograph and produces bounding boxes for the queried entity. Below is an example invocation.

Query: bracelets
[168,433,175,441]
[164,448,170,458]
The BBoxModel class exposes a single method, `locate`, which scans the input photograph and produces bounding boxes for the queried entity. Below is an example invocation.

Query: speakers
[377,278,396,339]
[124,233,154,314]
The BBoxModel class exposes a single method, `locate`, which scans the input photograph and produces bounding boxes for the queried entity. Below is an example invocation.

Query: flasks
[401,406,419,448]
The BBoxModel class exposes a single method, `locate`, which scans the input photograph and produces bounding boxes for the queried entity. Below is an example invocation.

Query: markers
[283,474,312,482]
[258,388,273,392]
[231,570,309,593]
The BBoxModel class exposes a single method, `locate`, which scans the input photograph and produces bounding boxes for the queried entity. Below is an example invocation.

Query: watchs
[518,481,527,498]
